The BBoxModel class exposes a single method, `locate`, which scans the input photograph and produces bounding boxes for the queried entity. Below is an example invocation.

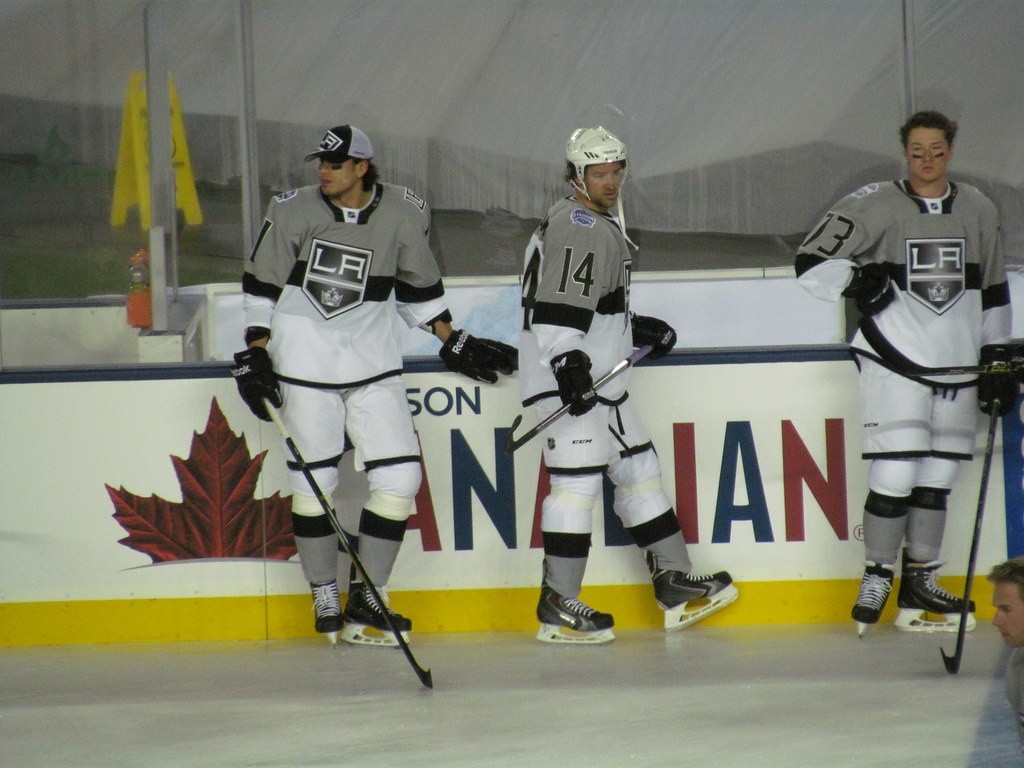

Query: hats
[304,125,373,162]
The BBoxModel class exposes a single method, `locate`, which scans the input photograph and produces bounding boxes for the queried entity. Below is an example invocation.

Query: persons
[232,126,518,649]
[794,110,1013,638]
[987,557,1024,754]
[520,127,738,646]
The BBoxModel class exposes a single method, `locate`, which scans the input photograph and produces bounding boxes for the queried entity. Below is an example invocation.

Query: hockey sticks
[858,316,1024,377]
[503,340,654,454]
[261,395,434,689]
[940,398,1001,674]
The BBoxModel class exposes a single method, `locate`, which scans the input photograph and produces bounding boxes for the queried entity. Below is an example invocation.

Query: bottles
[128,249,150,294]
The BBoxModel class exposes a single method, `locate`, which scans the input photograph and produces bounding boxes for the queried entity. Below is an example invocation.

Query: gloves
[841,269,895,316]
[632,316,677,357]
[438,329,518,384]
[978,343,1017,415]
[550,350,598,416]
[231,346,282,423]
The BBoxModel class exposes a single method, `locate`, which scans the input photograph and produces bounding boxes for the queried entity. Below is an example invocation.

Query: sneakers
[342,562,412,646]
[642,550,738,633]
[309,579,342,643]
[537,559,616,646]
[895,547,976,632]
[851,562,895,639]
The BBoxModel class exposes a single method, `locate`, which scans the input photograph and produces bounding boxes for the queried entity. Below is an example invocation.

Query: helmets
[566,126,626,179]
